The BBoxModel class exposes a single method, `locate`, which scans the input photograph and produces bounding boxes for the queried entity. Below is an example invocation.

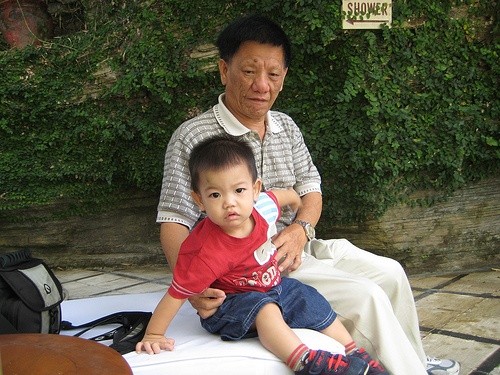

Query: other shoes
[294,349,369,375]
[425,357,460,375]
[348,348,389,375]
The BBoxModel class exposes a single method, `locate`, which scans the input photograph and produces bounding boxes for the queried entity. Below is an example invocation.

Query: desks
[0,334,134,375]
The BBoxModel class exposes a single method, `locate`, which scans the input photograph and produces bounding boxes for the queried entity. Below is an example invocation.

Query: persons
[156,18,461,375]
[135,134,390,375]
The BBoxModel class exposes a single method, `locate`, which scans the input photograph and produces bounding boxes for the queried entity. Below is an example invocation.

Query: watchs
[292,219,316,241]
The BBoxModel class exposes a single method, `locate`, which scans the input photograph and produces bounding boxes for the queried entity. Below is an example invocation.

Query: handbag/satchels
[0,248,65,335]
